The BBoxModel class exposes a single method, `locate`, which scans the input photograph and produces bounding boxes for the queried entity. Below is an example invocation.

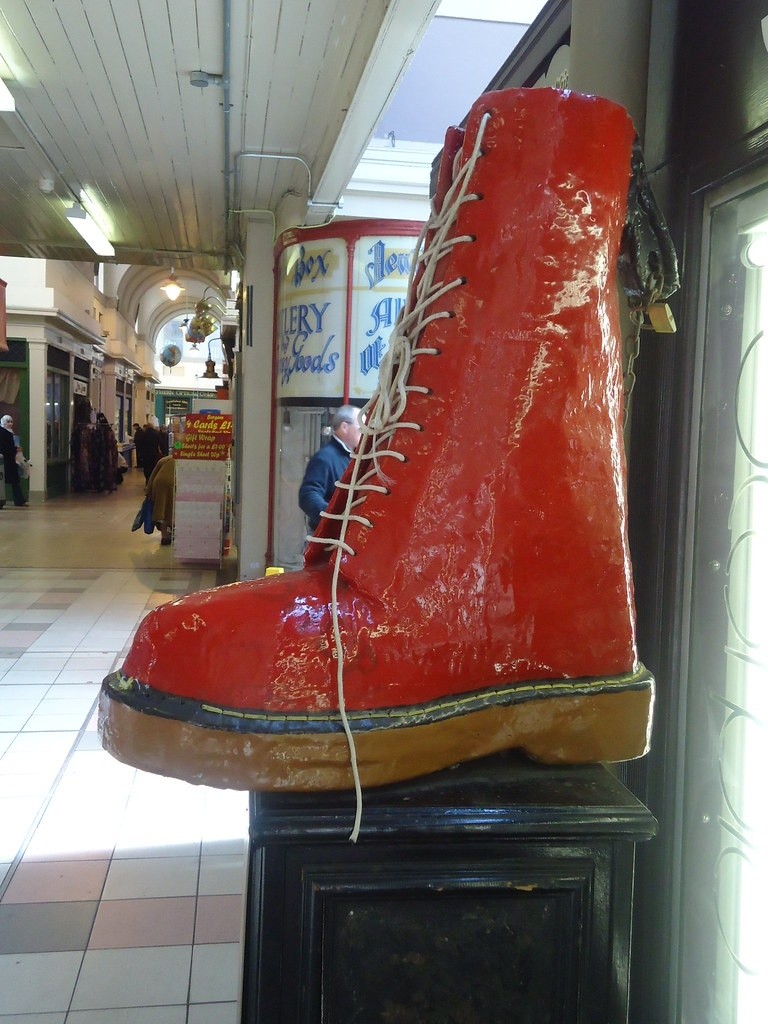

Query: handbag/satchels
[131,500,145,531]
[143,495,154,534]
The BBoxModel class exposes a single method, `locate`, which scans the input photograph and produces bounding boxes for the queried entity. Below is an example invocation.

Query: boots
[100,86,654,842]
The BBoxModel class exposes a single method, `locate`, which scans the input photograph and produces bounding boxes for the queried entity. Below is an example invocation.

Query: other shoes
[15,502,30,507]
[134,465,141,468]
[161,533,171,546]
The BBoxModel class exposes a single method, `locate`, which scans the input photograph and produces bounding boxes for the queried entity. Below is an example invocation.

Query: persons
[132,422,170,487]
[0,415,30,507]
[296,405,367,537]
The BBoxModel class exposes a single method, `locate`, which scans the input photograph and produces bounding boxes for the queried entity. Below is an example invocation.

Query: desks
[242,748,659,1024]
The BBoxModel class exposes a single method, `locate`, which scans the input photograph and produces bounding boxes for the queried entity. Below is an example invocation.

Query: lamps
[321,407,332,436]
[64,203,116,256]
[159,267,185,301]
[0,76,15,112]
[189,285,226,336]
[282,407,293,432]
